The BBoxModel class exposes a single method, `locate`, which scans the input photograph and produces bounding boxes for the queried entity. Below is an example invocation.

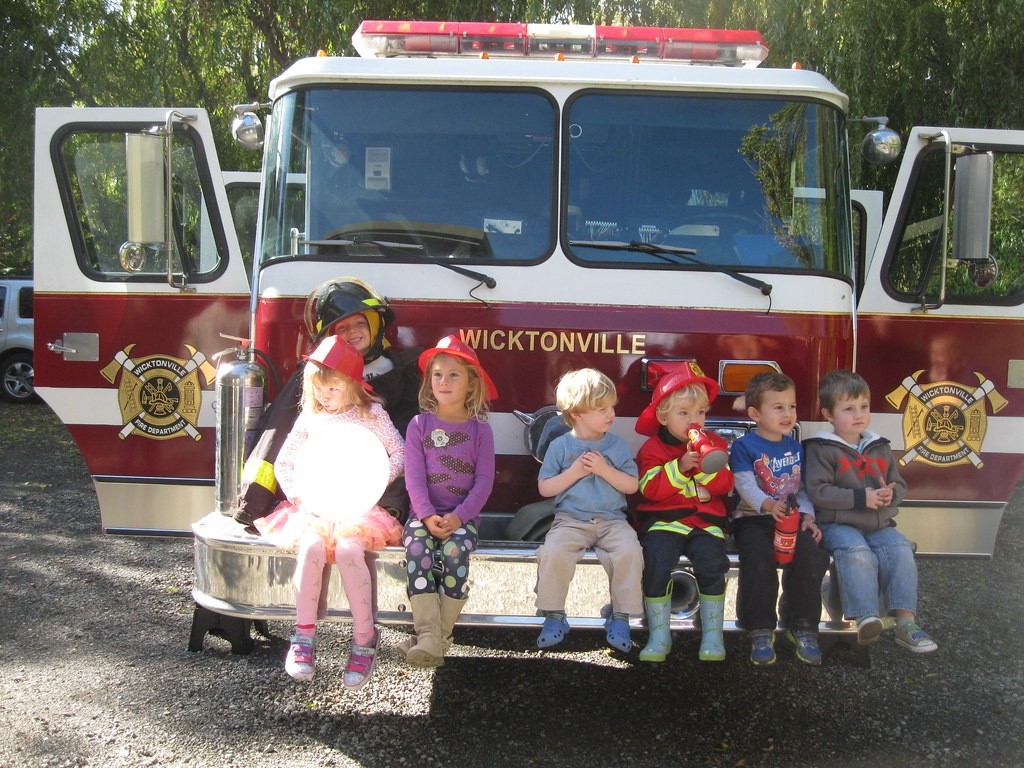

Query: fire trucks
[33,19,1024,633]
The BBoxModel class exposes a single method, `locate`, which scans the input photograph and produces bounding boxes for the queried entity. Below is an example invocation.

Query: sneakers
[894,621,938,652]
[285,634,316,682]
[857,614,884,647]
[784,626,822,667]
[749,628,776,665]
[343,626,380,690]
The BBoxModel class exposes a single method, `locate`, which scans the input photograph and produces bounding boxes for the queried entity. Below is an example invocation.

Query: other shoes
[537,618,571,648]
[603,614,631,652]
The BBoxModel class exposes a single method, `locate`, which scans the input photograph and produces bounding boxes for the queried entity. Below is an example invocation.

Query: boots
[406,593,445,670]
[694,578,728,661]
[639,578,673,661]
[397,593,469,658]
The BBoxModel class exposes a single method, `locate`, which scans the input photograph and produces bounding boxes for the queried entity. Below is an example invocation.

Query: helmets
[302,334,378,397]
[634,360,720,436]
[418,335,499,401]
[304,276,395,343]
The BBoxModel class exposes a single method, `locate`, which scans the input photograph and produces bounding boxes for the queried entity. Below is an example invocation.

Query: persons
[803,371,938,653]
[274,335,406,690]
[535,368,645,651]
[635,361,734,661]
[234,276,422,529]
[730,373,828,666]
[402,335,499,667]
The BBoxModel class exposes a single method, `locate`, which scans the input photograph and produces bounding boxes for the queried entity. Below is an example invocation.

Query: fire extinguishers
[212,331,282,518]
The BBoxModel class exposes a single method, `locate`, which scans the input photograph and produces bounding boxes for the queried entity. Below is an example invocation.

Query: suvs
[0,276,44,402]
